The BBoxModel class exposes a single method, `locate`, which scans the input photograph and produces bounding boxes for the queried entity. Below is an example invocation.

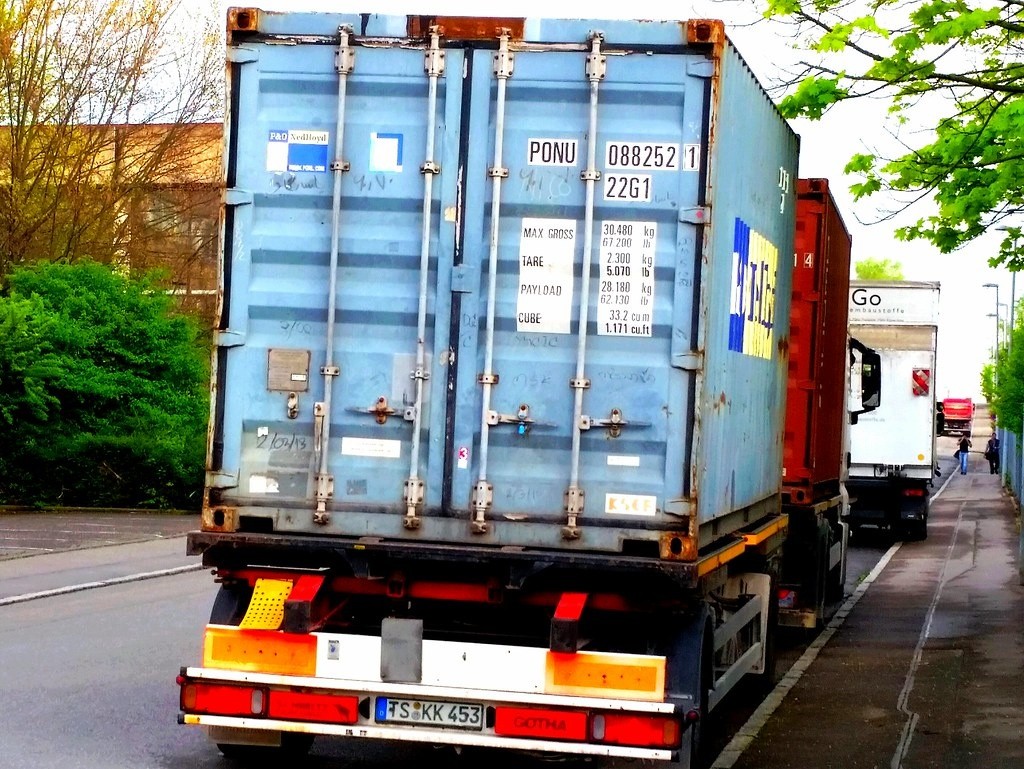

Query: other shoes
[995,470,998,475]
[961,472,966,475]
[991,471,994,474]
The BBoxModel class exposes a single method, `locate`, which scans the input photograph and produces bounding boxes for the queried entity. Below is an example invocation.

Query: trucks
[941,397,975,438]
[778,178,881,643]
[845,280,946,544]
[174,6,801,769]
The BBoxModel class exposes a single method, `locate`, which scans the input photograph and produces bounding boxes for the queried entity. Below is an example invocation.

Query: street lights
[983,283,999,440]
[996,303,1008,349]
[986,302,999,384]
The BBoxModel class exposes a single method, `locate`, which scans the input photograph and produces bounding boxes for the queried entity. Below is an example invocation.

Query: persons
[957,434,972,476]
[983,433,1001,474]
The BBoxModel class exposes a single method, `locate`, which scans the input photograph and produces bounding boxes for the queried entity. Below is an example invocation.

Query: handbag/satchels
[985,452,990,460]
